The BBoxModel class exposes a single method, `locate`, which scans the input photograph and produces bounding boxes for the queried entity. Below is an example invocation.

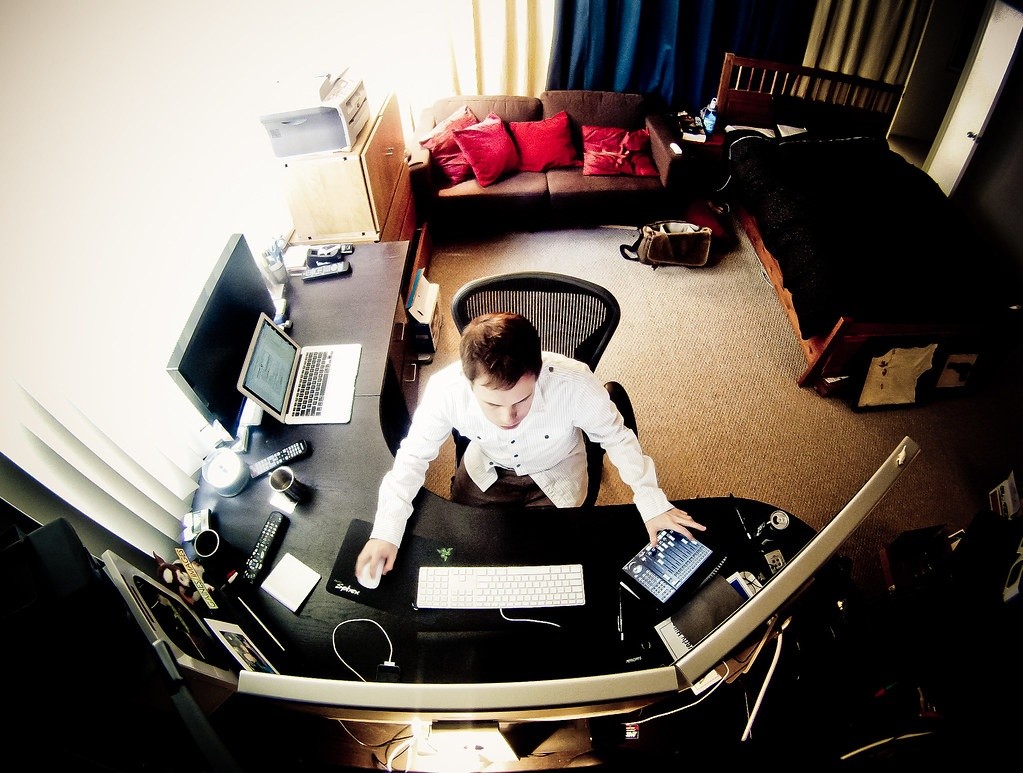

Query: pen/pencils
[617,588,625,641]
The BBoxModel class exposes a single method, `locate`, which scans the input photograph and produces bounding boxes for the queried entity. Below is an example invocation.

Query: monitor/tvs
[238,436,921,763]
[166,232,277,442]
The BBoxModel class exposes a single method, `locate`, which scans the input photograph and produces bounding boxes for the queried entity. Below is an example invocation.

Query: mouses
[357,559,385,589]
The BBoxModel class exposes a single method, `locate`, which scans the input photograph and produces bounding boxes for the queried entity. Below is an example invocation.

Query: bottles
[703,97,717,140]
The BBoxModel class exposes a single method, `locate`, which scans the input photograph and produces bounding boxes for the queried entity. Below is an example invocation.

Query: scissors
[272,239,286,262]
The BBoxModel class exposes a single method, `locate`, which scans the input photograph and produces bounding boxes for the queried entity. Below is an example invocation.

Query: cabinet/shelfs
[279,89,432,311]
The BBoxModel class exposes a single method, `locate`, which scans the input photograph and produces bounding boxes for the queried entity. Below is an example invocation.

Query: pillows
[451,110,520,189]
[418,105,481,185]
[507,111,584,175]
[579,124,660,178]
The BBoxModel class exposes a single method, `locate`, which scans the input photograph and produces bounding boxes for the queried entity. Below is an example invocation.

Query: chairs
[451,269,639,507]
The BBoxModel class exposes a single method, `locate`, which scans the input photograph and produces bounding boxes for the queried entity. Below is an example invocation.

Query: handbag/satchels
[620,220,712,271]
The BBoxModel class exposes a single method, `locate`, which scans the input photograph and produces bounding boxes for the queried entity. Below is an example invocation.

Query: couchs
[433,88,682,228]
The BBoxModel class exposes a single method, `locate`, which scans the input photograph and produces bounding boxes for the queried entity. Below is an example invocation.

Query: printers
[258,64,371,157]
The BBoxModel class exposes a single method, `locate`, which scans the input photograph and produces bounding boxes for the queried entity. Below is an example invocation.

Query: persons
[354,313,706,578]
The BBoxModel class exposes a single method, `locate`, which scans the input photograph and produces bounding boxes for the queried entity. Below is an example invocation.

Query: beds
[715,53,1021,388]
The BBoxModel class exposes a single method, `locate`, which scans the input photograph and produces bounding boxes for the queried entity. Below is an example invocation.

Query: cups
[269,262,291,288]
[194,529,233,567]
[269,466,309,504]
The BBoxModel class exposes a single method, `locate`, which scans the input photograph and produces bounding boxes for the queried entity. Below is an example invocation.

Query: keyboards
[416,564,585,609]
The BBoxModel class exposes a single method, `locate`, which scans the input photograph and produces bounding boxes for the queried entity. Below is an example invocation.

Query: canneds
[756,511,790,545]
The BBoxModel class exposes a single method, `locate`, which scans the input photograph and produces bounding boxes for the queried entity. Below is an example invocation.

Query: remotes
[242,510,286,585]
[248,439,311,479]
[300,262,350,281]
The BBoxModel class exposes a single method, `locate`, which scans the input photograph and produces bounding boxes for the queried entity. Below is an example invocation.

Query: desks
[172,238,823,712]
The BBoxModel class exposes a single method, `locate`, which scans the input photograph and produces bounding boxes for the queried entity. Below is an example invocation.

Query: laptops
[237,312,362,425]
